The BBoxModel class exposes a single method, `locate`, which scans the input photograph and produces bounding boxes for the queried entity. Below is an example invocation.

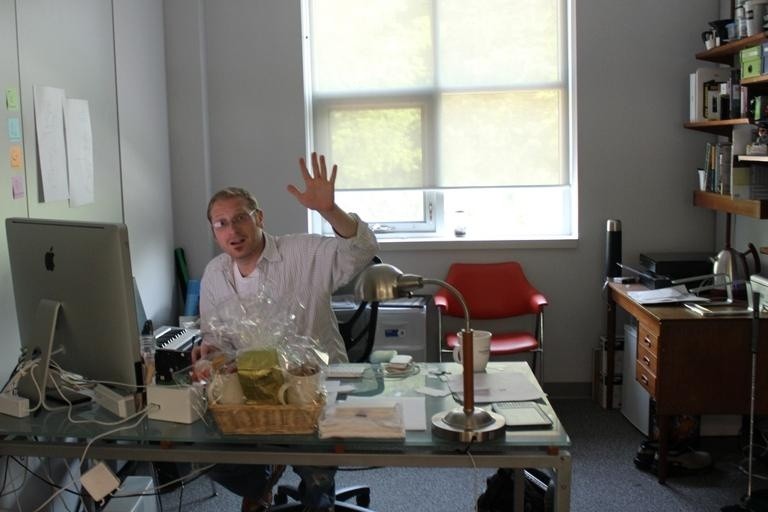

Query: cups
[210,373,246,404]
[744,4,764,38]
[277,366,324,406]
[453,329,492,373]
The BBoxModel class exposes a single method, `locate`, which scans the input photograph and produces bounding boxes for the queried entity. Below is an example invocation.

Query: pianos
[153,325,202,353]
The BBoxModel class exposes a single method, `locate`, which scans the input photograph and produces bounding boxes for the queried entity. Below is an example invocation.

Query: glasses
[213,208,257,230]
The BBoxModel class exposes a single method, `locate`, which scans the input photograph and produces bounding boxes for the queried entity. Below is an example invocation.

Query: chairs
[259,247,386,512]
[433,260,552,391]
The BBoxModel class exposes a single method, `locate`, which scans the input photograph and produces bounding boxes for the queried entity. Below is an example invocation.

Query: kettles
[711,244,761,298]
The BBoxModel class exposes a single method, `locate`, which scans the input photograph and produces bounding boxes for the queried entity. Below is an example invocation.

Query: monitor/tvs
[5,214,143,409]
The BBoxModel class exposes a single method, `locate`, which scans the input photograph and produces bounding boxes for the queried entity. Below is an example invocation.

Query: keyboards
[323,362,368,377]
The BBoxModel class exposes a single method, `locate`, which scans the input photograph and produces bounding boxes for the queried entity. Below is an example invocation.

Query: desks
[606,276,768,480]
[0,362,576,511]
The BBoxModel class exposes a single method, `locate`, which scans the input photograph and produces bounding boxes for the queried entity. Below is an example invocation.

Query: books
[689,68,740,123]
[704,142,731,195]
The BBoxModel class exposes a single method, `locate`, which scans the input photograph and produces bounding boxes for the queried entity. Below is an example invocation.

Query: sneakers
[241,489,273,512]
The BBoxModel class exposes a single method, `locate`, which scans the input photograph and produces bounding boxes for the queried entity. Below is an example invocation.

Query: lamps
[356,264,508,445]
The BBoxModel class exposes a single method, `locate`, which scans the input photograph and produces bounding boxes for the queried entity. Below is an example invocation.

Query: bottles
[605,218,624,282]
[454,209,468,238]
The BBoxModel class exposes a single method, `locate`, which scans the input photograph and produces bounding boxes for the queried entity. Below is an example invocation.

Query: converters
[80,461,122,501]
[0,393,30,420]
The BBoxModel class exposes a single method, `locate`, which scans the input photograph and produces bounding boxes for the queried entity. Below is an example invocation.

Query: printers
[617,251,715,290]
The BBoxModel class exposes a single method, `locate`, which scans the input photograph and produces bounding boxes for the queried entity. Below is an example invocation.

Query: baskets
[208,399,325,435]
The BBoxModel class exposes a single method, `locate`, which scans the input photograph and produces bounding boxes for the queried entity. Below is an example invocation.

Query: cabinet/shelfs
[689,30,768,220]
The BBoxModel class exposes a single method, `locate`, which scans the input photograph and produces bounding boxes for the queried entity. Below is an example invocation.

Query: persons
[190,152,379,512]
